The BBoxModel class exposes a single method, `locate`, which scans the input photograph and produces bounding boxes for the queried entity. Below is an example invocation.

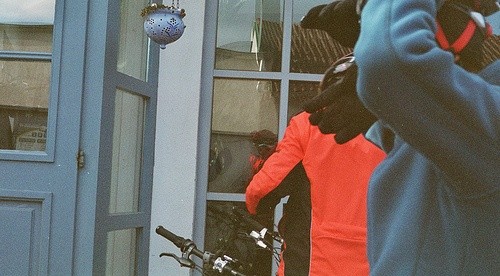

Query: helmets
[316,52,355,92]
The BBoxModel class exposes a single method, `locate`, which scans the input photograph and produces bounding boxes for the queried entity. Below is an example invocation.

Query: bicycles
[152,206,283,276]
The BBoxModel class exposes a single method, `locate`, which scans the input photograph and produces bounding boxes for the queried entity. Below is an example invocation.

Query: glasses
[253,142,276,156]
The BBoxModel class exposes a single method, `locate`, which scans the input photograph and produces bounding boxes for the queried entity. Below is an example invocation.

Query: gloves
[302,50,376,146]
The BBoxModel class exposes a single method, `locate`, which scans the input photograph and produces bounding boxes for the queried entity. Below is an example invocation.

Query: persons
[298,1,500,276]
[244,91,387,276]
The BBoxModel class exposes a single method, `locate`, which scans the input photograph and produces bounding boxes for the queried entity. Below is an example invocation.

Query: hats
[249,127,277,158]
